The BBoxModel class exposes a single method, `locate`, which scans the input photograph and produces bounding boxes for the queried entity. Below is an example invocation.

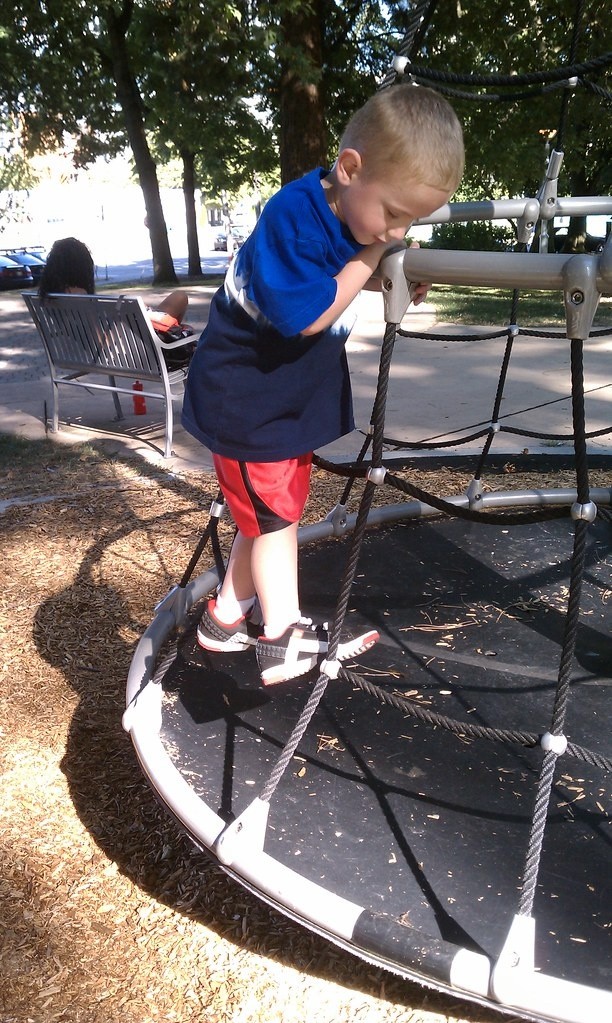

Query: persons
[37,237,188,363]
[180,83,466,686]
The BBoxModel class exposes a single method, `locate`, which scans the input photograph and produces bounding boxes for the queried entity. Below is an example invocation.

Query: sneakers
[196,599,270,653]
[255,618,385,687]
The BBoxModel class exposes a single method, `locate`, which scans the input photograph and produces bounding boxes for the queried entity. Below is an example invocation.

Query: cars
[5,252,46,286]
[29,251,49,263]
[0,255,34,291]
[214,234,228,251]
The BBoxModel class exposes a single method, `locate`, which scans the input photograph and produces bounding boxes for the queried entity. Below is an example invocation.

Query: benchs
[22,291,209,459]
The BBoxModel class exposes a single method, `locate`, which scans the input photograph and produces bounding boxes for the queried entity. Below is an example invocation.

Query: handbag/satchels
[153,326,197,372]
